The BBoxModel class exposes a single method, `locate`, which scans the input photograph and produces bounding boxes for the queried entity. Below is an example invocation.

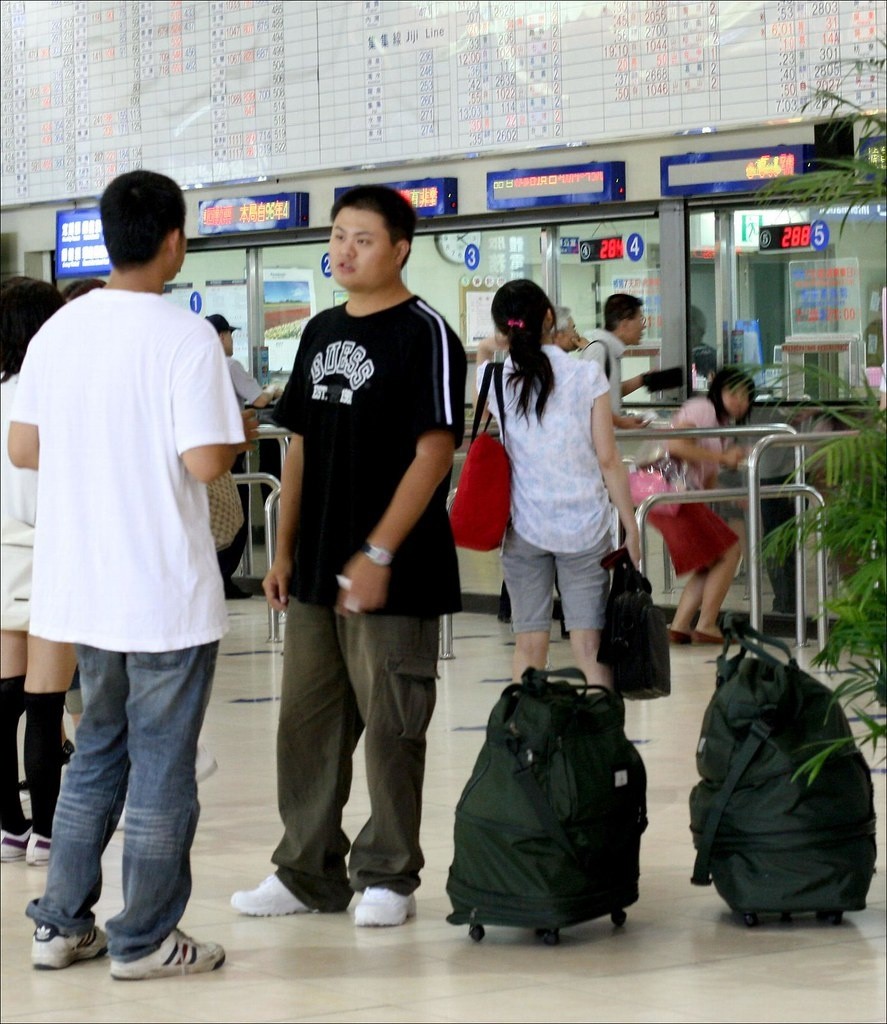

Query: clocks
[434,231,482,266]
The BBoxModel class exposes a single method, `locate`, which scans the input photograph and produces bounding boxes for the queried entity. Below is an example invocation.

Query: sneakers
[33,921,111,969]
[231,871,311,916]
[354,885,418,926]
[111,925,226,979]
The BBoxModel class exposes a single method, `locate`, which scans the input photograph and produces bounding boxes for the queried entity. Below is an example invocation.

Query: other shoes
[0,829,33,862]
[671,621,735,647]
[25,831,58,865]
[222,582,251,603]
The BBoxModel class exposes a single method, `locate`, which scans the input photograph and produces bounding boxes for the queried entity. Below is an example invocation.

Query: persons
[556,291,652,641]
[198,314,280,598]
[231,183,468,930]
[498,303,590,624]
[0,276,67,865]
[655,366,757,646]
[475,278,640,698]
[8,171,228,979]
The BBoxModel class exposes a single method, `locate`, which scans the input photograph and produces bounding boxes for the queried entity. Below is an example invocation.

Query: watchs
[362,541,392,566]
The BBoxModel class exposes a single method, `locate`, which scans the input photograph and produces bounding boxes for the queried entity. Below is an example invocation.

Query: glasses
[627,316,646,324]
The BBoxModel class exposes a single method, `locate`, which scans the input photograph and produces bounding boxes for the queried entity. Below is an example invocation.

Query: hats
[205,314,239,332]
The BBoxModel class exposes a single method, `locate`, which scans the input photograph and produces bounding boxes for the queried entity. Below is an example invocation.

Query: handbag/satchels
[205,468,245,551]
[448,362,510,551]
[597,551,670,700]
[626,456,690,516]
[689,612,876,927]
[447,663,647,947]
